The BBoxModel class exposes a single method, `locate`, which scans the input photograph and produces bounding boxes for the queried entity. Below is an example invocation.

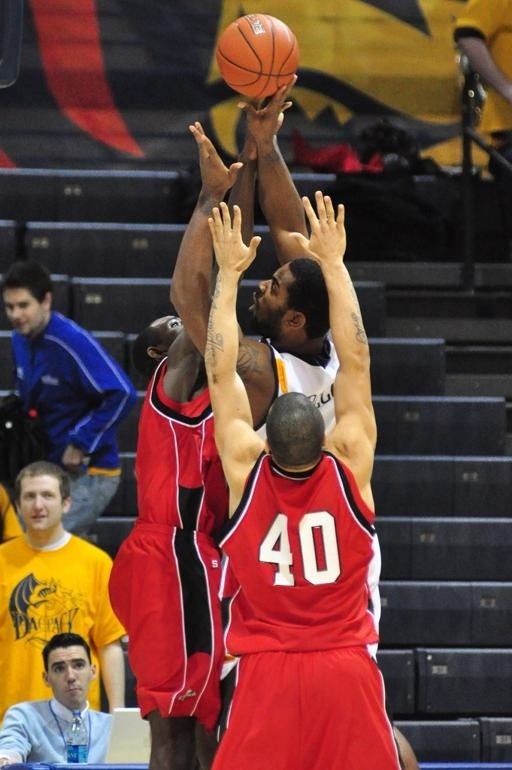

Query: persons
[0,459,129,729]
[109,73,419,769]
[451,0,512,178]
[0,631,113,768]
[0,259,138,531]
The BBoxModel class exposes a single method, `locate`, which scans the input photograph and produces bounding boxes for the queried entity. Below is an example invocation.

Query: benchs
[0,164,512,770]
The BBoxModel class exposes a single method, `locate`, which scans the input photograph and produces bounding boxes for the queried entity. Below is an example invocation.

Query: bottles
[65,711,88,764]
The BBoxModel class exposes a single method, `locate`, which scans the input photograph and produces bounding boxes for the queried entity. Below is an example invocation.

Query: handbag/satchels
[1,393,50,483]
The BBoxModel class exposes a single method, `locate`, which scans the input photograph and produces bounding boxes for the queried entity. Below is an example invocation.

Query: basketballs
[217,14,299,98]
